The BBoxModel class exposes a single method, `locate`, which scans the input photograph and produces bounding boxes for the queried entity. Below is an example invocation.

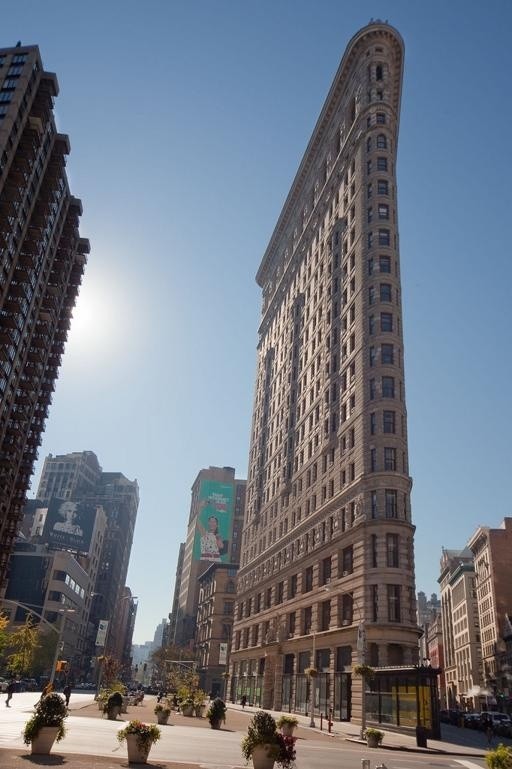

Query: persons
[483,716,493,744]
[61,682,74,706]
[4,679,16,708]
[240,692,246,708]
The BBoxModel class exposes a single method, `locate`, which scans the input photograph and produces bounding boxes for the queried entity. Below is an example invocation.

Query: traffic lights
[499,692,505,701]
[56,662,68,671]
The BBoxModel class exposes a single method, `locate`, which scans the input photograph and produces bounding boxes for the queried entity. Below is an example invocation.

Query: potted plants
[24,692,67,755]
[364,728,385,748]
[154,686,227,729]
[96,680,145,719]
[240,710,300,769]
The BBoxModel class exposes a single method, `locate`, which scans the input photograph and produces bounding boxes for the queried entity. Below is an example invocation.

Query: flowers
[111,720,161,762]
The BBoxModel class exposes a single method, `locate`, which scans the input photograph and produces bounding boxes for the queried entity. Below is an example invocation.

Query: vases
[126,732,152,762]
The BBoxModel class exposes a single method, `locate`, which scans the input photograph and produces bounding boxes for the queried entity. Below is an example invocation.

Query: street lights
[41,607,76,691]
[90,587,138,700]
[320,582,364,666]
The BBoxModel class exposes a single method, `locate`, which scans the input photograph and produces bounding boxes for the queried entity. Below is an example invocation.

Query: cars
[440,710,511,737]
[0,674,159,695]
